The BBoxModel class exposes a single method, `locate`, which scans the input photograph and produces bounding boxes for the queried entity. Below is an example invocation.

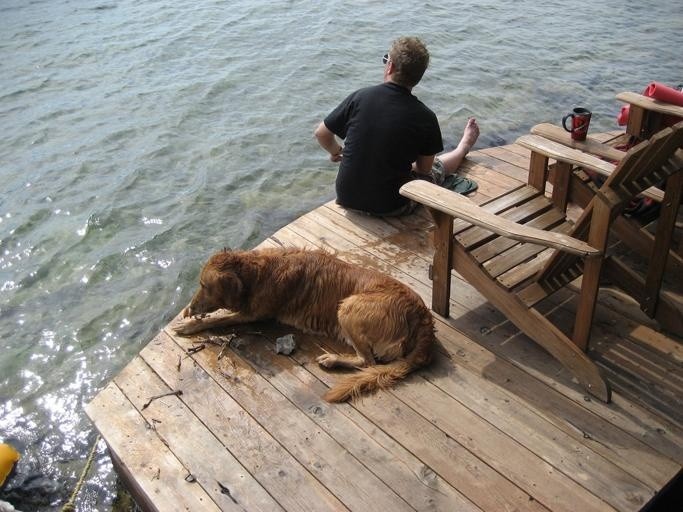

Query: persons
[313,35,478,216]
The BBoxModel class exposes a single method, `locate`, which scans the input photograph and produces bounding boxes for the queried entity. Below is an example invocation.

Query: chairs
[396,120,681,402]
[527,84,681,282]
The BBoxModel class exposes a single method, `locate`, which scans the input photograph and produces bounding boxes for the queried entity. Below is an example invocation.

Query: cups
[559,106,592,141]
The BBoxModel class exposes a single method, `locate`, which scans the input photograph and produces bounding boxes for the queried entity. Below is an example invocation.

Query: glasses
[382,55,394,68]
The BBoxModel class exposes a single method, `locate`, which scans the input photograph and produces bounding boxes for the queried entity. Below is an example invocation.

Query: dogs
[171,245,439,406]
